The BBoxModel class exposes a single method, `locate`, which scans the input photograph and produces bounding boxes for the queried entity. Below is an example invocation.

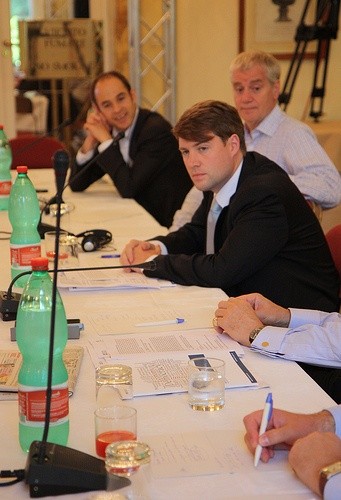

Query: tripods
[279,0,341,123]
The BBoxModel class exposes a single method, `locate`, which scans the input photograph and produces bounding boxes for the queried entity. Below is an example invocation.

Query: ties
[206,198,222,255]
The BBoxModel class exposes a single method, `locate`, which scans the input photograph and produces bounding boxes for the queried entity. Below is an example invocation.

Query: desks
[0,167,341,500]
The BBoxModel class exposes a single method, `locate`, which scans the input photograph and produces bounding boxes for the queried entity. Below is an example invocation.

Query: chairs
[4,131,68,171]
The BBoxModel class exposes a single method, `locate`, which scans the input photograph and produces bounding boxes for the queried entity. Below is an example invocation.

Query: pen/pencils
[255,393,273,466]
[134,317,184,327]
[100,254,120,258]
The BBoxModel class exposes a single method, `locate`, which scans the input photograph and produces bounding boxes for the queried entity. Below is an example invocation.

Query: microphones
[0,119,155,322]
[24,151,108,500]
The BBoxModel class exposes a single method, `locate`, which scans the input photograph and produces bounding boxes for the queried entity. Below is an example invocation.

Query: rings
[212,317,217,327]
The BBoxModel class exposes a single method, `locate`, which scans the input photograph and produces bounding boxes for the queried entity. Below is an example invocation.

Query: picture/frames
[236,0,330,61]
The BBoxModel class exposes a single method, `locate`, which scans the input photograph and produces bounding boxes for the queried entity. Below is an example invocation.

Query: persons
[243,404,341,500]
[165,51,341,233]
[121,100,341,404]
[213,293,341,370]
[69,71,193,229]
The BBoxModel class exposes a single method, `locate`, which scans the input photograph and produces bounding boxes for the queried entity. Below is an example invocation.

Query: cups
[44,231,68,259]
[96,365,134,401]
[105,441,153,499]
[188,356,228,410]
[94,404,137,458]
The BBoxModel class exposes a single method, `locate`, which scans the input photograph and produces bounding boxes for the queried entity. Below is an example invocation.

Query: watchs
[249,326,265,345]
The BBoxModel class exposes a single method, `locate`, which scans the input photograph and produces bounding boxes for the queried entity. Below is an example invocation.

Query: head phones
[76,229,113,252]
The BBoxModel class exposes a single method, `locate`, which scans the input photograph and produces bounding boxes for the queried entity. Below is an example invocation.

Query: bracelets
[319,461,341,500]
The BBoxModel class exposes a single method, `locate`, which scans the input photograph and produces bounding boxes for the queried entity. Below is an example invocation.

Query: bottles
[0,123,13,210]
[17,259,70,453]
[10,166,43,285]
